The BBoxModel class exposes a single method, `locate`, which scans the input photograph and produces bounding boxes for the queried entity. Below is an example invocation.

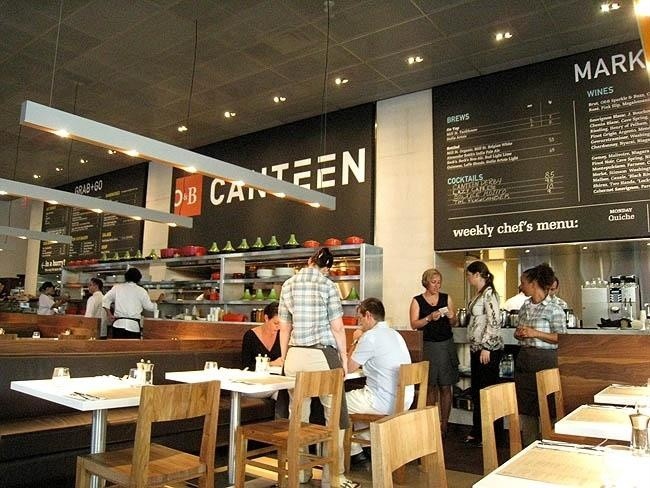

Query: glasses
[359,301,368,313]
[89,284,94,287]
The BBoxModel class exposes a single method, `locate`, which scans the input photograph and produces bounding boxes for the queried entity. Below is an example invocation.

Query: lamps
[0,15,213,229]
[0,83,82,247]
[18,0,379,211]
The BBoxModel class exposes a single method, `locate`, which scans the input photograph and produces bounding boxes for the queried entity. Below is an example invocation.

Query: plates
[225,273,233,278]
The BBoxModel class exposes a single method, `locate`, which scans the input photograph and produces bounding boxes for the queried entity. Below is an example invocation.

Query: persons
[278,247,360,488]
[38,281,68,315]
[102,267,164,339]
[549,277,567,310]
[241,302,290,419]
[85,277,109,340]
[505,285,527,312]
[346,298,416,467]
[460,261,505,444]
[410,269,459,438]
[513,264,566,446]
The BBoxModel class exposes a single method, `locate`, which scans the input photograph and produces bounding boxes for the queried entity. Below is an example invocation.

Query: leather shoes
[463,433,481,441]
[350,452,371,472]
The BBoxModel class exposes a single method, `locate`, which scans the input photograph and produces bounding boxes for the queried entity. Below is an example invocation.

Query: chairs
[535,368,611,446]
[368,406,448,488]
[344,359,429,476]
[234,367,346,487]
[478,382,523,477]
[74,380,222,487]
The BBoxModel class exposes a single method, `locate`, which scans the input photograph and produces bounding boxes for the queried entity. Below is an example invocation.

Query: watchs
[424,316,431,322]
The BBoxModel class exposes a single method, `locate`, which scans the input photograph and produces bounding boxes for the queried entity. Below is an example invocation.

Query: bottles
[192,302,199,321]
[261,354,270,378]
[254,353,265,378]
[250,308,256,321]
[184,306,189,322]
[145,359,154,387]
[629,410,649,453]
[136,359,145,384]
[506,354,514,375]
[256,309,263,320]
[645,305,650,330]
[498,358,503,379]
[501,355,507,376]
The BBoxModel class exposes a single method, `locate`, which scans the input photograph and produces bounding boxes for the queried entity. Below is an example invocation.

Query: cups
[51,367,71,395]
[210,291,217,300]
[629,413,650,456]
[129,369,138,389]
[32,331,40,338]
[247,272,256,279]
[204,361,218,381]
[233,273,242,279]
[455,307,466,326]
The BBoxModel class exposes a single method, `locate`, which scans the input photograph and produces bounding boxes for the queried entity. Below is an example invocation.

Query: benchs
[0,350,277,488]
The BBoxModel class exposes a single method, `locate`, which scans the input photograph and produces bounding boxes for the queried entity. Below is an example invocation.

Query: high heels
[442,427,449,440]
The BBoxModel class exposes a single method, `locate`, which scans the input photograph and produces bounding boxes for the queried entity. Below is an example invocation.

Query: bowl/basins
[180,246,206,257]
[275,268,294,278]
[257,269,273,278]
[160,248,180,258]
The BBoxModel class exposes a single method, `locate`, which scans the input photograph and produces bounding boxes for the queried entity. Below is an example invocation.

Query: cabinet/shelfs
[60,243,383,326]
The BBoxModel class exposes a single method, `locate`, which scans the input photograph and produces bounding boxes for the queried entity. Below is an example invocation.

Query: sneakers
[340,477,361,488]
[301,475,313,484]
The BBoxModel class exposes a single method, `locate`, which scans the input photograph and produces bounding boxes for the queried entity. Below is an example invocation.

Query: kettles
[563,308,577,328]
